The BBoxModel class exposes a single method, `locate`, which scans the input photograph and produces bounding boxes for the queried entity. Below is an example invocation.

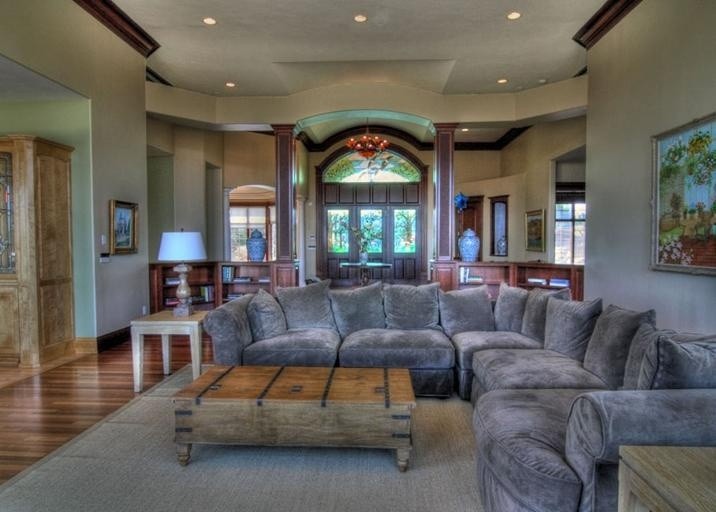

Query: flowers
[350,216,384,252]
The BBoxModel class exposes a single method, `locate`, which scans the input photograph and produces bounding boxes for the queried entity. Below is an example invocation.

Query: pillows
[274,278,336,330]
[522,286,572,343]
[494,282,525,331]
[328,281,386,339]
[246,287,287,342]
[622,322,704,389]
[544,295,603,362]
[582,303,656,389]
[650,334,714,388]
[382,282,443,332]
[435,285,494,338]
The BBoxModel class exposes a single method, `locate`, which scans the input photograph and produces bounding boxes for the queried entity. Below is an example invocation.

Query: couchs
[203,293,716,512]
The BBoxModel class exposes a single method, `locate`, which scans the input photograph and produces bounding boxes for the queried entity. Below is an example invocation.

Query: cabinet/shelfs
[216,263,273,306]
[510,263,583,303]
[0,133,74,367]
[152,262,214,313]
[458,262,510,305]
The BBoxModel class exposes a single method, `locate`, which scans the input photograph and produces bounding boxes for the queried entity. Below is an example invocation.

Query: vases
[457,228,480,262]
[246,229,267,261]
[358,250,369,265]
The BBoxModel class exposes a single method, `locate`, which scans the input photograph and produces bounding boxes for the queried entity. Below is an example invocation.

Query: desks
[339,262,392,289]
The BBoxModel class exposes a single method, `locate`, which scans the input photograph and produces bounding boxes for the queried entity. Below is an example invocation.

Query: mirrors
[488,195,509,257]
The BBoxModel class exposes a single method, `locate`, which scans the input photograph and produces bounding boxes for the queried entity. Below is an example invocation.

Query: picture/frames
[524,209,545,252]
[649,112,716,276]
[109,199,139,256]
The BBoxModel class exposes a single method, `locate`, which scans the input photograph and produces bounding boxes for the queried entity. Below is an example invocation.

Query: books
[549,278,570,287]
[227,294,244,299]
[460,267,483,284]
[222,266,252,282]
[528,278,548,286]
[200,285,214,302]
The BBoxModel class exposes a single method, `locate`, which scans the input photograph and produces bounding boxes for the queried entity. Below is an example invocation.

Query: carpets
[1,362,485,512]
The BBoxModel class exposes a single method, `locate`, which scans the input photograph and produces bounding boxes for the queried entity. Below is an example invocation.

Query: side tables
[131,311,211,393]
[616,444,715,512]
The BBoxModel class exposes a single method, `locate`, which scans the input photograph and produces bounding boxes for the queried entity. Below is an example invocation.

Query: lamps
[158,228,209,316]
[344,118,389,158]
